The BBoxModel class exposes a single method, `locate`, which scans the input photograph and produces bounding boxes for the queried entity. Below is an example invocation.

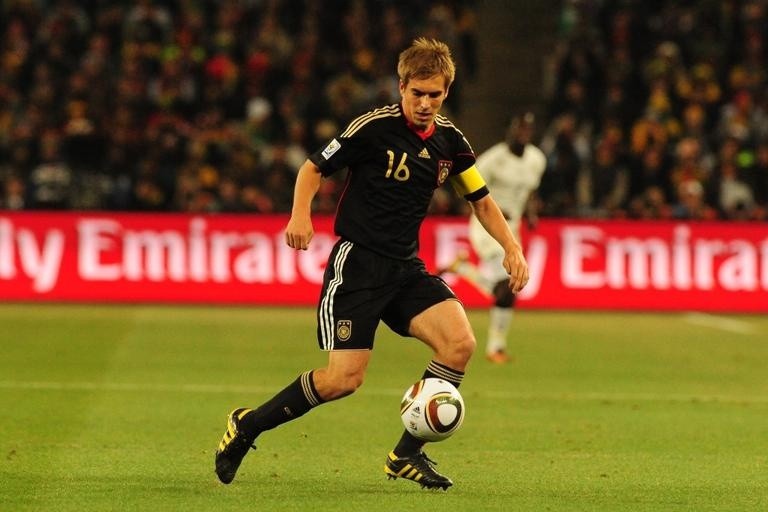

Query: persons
[2,1,478,215]
[430,110,548,366]
[535,1,767,223]
[212,32,534,492]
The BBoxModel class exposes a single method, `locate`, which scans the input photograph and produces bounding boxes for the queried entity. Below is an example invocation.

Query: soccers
[401,378,465,442]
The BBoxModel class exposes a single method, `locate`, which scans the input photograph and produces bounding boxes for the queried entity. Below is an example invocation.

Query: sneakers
[383,449,453,491]
[216,407,257,484]
[485,346,515,364]
